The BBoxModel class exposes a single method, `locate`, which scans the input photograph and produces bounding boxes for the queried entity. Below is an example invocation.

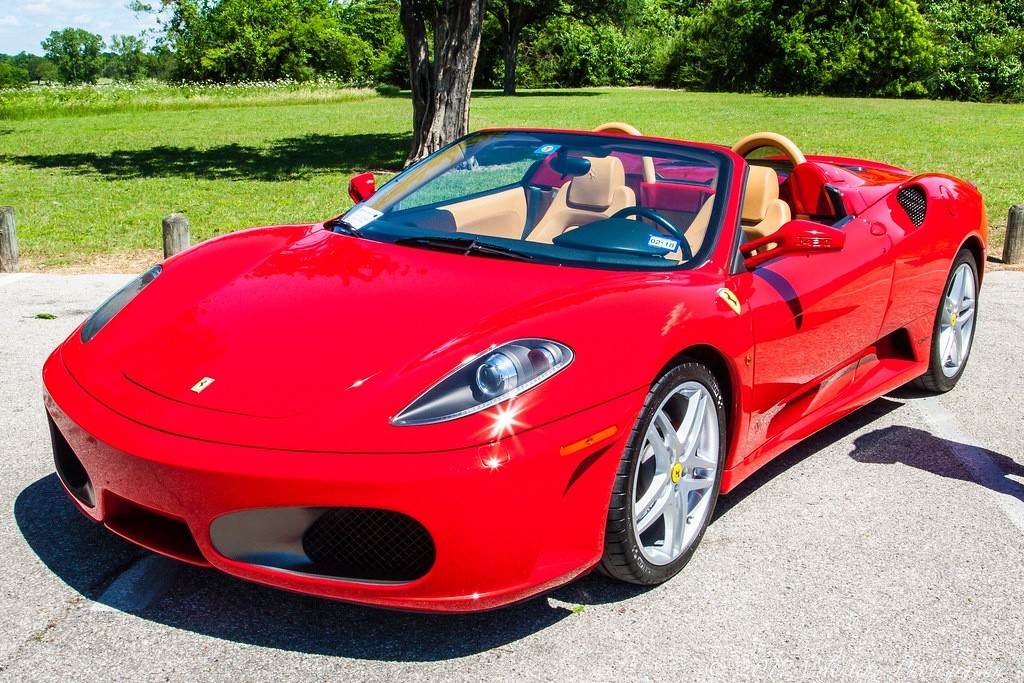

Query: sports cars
[42,118,989,615]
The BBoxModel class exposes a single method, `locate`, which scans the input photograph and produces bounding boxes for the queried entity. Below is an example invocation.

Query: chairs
[711,131,806,192]
[594,121,654,185]
[666,167,788,263]
[525,152,635,244]
